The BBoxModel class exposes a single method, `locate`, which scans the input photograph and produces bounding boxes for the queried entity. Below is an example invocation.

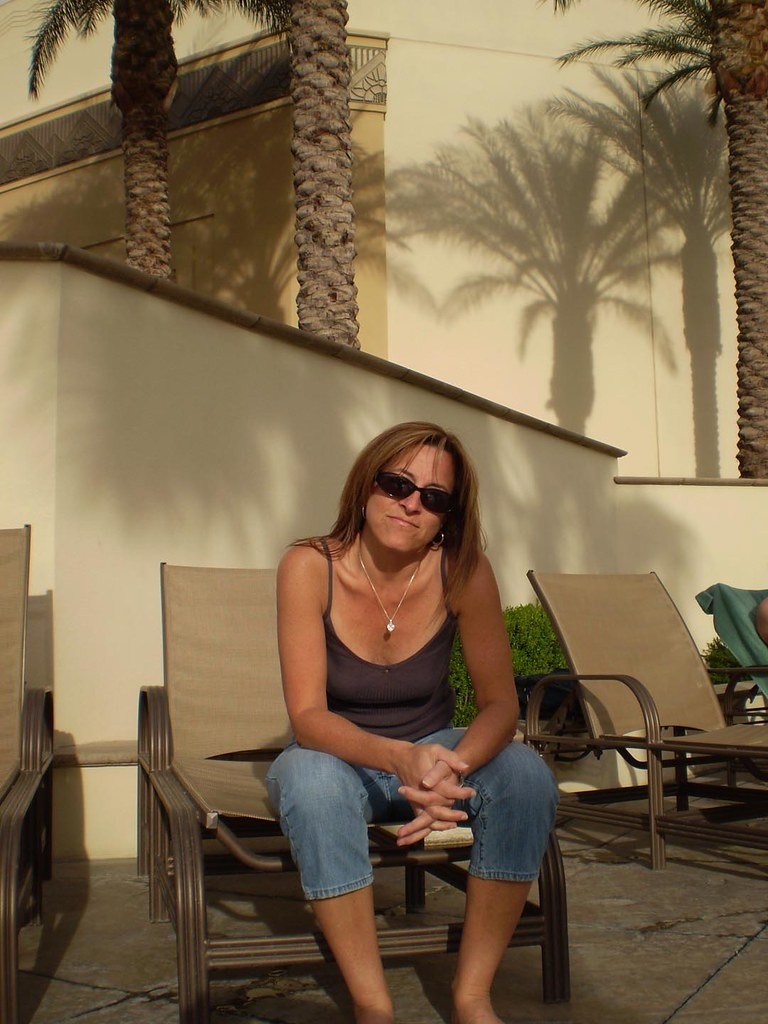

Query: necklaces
[359,551,422,632]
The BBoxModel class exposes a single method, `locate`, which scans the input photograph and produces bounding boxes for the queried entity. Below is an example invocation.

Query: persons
[264,421,561,1024]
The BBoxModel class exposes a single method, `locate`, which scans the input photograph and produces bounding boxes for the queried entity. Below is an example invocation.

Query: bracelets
[458,770,465,788]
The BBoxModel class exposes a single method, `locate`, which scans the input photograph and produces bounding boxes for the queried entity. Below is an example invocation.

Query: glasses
[375,469,456,513]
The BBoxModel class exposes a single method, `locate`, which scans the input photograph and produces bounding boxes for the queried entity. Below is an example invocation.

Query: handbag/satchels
[514,669,582,719]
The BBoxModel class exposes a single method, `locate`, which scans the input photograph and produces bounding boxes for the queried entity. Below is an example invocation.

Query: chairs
[136,560,572,1024]
[523,569,768,871]
[695,583,768,782]
[0,523,56,1024]
